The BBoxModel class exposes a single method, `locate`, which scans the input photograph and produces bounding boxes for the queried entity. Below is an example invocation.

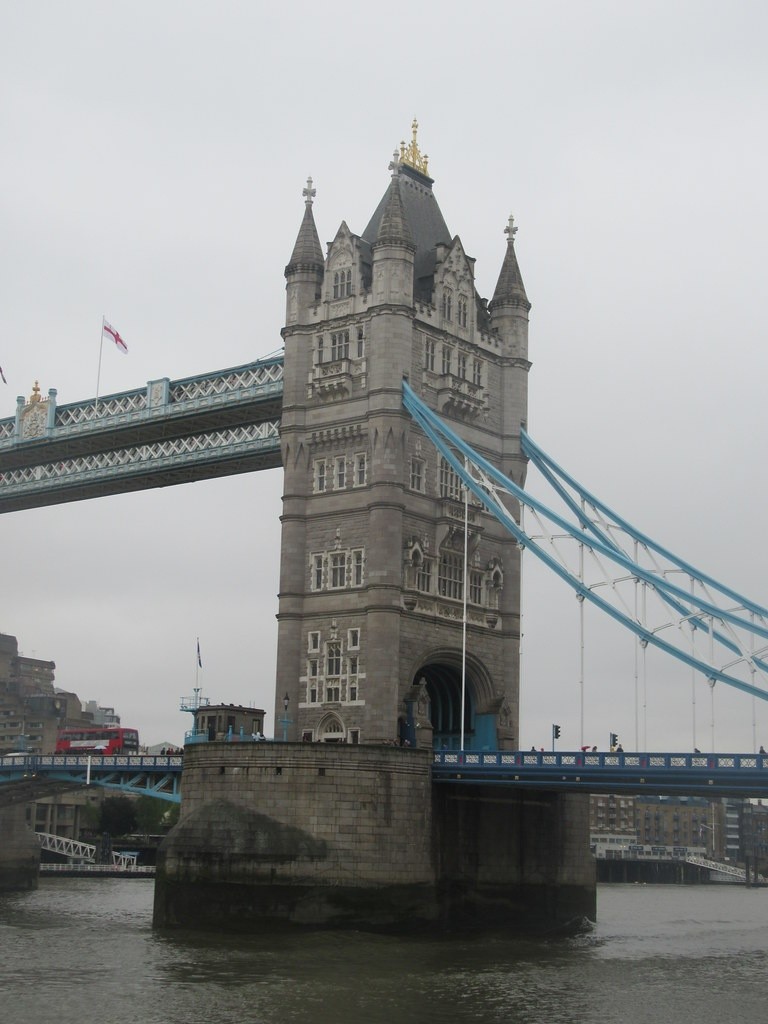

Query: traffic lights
[557,726,560,737]
[615,735,618,745]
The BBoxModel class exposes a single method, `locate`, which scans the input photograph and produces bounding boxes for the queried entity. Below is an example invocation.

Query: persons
[759,746,767,754]
[531,746,536,751]
[591,746,598,752]
[616,744,623,752]
[442,743,449,755]
[159,747,184,755]
[694,747,701,754]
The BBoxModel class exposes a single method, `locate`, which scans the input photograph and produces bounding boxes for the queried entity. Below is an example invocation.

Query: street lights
[283,692,289,740]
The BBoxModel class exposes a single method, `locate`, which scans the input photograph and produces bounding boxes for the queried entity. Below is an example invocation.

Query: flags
[103,319,129,355]
[198,643,203,668]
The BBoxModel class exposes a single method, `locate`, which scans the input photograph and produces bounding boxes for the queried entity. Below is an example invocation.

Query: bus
[55,728,139,756]
